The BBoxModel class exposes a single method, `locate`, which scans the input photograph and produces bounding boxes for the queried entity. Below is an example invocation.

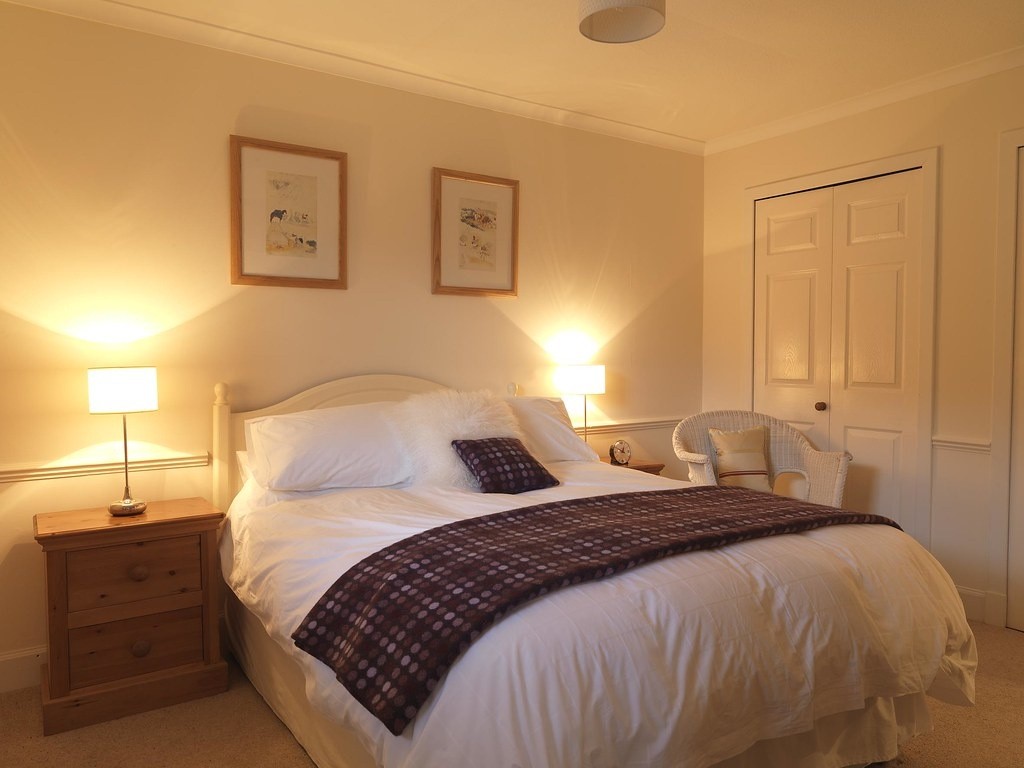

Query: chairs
[672,409,853,510]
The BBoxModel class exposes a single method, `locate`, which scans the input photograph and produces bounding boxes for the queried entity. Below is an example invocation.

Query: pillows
[452,435,561,494]
[707,426,773,494]
[772,468,811,501]
[243,400,416,492]
[392,388,538,493]
[504,397,601,464]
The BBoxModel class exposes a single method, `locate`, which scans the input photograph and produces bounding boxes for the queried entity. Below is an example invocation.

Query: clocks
[608,439,631,466]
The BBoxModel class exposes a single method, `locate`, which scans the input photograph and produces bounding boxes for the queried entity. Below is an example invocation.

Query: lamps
[556,365,604,443]
[578,0,667,43]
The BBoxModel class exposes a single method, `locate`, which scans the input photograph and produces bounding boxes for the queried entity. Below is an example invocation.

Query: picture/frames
[228,134,348,289]
[431,167,520,298]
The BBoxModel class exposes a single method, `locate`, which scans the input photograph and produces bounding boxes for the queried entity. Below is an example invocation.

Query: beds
[212,374,978,768]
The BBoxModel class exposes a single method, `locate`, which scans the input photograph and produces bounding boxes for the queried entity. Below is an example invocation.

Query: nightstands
[88,366,158,516]
[32,496,231,736]
[599,456,666,476]
